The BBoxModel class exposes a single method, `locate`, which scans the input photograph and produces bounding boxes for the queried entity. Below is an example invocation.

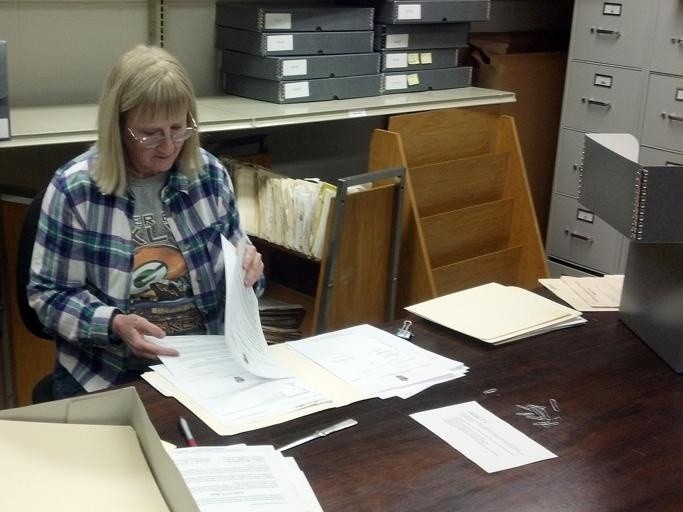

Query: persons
[28,42,267,401]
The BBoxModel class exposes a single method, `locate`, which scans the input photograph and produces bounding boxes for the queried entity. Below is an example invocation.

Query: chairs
[16,188,58,405]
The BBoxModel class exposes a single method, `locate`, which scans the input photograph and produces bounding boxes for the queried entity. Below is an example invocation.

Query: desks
[1,285,682,512]
[1,88,515,177]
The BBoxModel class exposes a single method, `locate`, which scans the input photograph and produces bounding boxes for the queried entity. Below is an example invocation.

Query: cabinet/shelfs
[371,106,551,319]
[545,0,682,282]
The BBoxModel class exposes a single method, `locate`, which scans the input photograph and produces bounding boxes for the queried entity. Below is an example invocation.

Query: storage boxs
[459,29,571,247]
[465,38,569,257]
[1,386,201,512]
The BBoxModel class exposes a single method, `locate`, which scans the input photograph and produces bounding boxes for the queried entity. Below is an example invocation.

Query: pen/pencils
[178,416,198,445]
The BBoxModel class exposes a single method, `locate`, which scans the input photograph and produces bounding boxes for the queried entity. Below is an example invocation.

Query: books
[379,71,473,95]
[215,154,368,260]
[221,50,382,82]
[167,443,324,512]
[143,233,286,394]
[375,0,490,22]
[380,49,460,70]
[288,323,470,403]
[401,282,589,347]
[216,26,374,55]
[250,291,306,349]
[376,24,471,48]
[224,72,383,106]
[538,272,628,315]
[215,1,375,31]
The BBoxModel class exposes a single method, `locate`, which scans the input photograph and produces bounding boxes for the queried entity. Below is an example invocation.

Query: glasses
[121,111,197,148]
